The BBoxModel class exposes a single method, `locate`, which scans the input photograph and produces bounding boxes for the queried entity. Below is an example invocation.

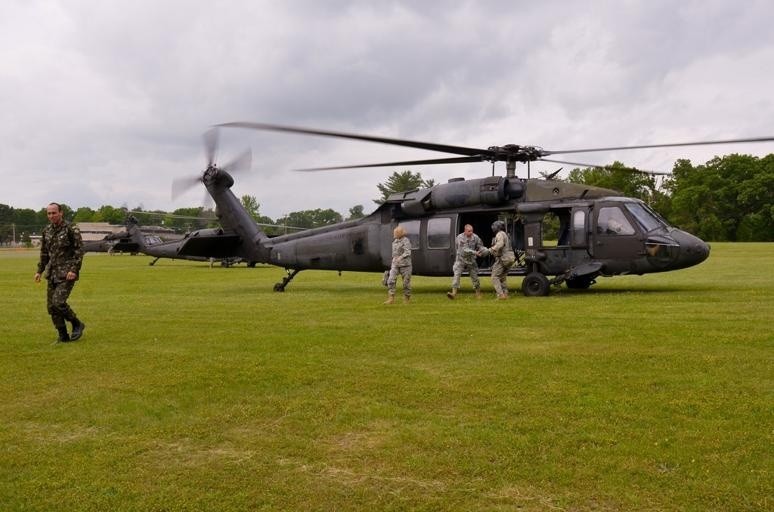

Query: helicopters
[157,118,774,302]
[70,226,165,257]
[119,214,280,270]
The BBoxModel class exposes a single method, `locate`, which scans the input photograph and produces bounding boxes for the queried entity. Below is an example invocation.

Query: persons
[382,226,413,306]
[32,202,85,345]
[446,223,485,301]
[487,220,516,300]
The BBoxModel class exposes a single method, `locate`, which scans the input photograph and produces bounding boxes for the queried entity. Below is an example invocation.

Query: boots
[55,327,69,344]
[403,296,409,305]
[70,318,84,340]
[476,289,480,298]
[383,295,395,304]
[448,288,458,300]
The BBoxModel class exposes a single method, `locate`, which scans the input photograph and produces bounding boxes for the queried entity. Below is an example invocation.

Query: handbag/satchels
[501,251,516,266]
[383,271,397,286]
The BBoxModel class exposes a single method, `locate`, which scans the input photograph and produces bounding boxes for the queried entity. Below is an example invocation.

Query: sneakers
[496,293,507,300]
